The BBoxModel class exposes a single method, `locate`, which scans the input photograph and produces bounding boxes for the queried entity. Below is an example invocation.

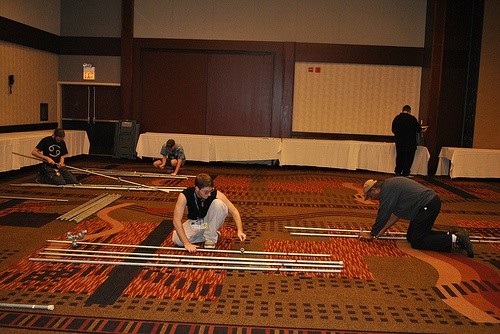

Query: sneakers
[456,233,474,258]
[449,228,460,234]
[204,239,215,249]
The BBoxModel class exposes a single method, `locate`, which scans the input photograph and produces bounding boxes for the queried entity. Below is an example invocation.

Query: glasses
[198,187,215,195]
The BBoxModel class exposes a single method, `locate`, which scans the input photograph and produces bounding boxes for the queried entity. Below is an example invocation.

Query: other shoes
[35,172,43,183]
[178,168,182,173]
[406,174,415,178]
[172,167,176,172]
[428,175,436,177]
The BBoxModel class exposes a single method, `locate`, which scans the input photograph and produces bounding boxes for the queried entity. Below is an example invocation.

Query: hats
[363,179,377,201]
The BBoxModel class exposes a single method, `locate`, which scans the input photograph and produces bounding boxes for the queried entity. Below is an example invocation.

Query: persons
[172,173,247,254]
[391,105,422,178]
[32,128,77,185]
[358,176,474,258]
[153,139,186,176]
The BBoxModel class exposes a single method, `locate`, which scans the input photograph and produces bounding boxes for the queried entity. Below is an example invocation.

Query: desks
[135,133,500,179]
[0,129,90,173]
